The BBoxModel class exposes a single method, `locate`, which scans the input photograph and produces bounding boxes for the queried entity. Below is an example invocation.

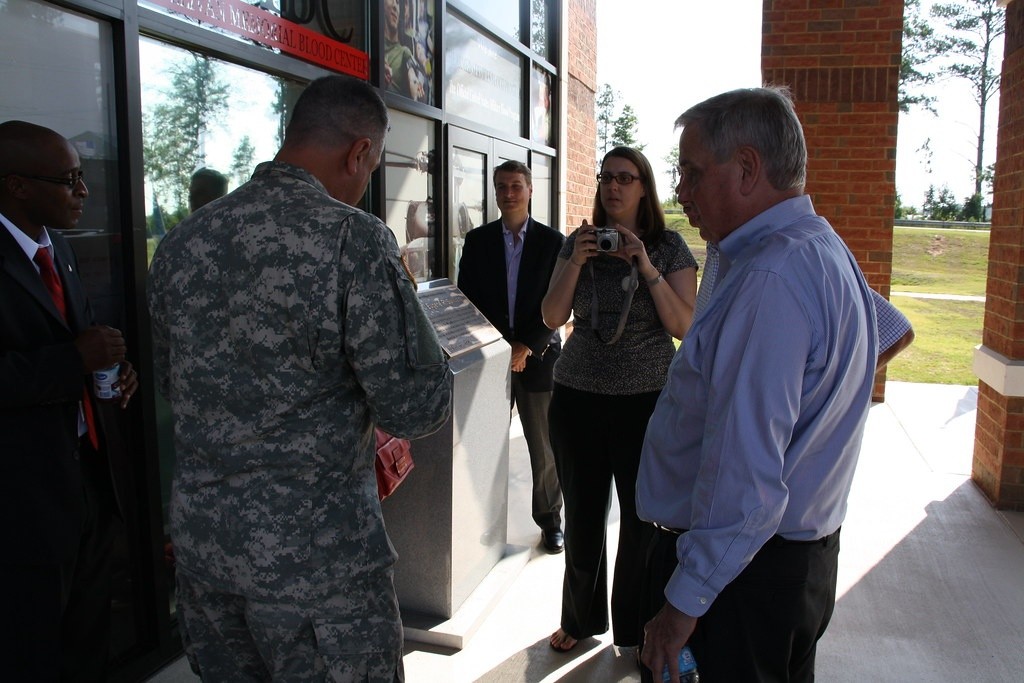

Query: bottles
[661,644,699,683]
[93,358,123,403]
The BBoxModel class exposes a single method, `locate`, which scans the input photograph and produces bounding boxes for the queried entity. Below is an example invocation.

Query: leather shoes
[541,527,565,554]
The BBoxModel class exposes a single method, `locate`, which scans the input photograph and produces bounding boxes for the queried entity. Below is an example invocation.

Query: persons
[542,146,700,653]
[189,167,229,212]
[457,160,569,553]
[0,121,139,683]
[635,86,878,683]
[384,0,433,102]
[147,75,455,683]
[688,236,915,374]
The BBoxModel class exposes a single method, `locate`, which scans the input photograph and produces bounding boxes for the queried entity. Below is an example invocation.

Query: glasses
[2,170,83,189]
[597,172,645,185]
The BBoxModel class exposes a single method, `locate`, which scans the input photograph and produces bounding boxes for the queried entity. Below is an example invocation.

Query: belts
[652,521,683,536]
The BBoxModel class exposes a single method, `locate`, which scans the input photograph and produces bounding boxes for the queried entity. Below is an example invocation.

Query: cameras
[586,228,619,252]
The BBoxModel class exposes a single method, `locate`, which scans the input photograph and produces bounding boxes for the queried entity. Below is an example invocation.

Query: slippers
[551,628,578,652]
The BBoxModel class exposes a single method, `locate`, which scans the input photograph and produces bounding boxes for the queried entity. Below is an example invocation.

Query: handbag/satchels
[376,429,416,501]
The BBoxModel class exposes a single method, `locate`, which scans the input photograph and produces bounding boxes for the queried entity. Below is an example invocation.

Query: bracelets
[571,257,582,267]
[647,273,664,288]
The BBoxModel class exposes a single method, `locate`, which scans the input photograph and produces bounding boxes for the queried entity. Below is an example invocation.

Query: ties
[33,246,99,451]
